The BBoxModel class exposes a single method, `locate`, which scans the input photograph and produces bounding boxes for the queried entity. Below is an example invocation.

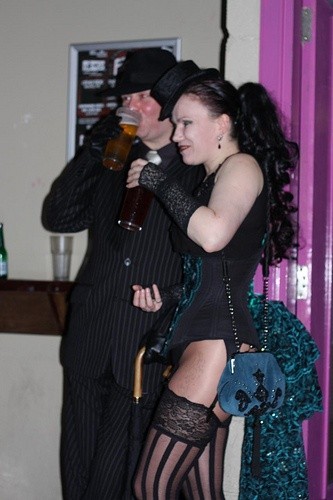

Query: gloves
[83,105,124,151]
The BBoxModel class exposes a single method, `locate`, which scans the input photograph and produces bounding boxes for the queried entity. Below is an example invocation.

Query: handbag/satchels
[217,351,286,417]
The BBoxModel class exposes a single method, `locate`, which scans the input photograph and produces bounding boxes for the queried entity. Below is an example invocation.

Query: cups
[50,236,74,281]
[100,106,143,170]
[117,185,153,233]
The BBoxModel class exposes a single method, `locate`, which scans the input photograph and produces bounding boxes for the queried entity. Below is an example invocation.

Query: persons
[126,59,301,500]
[41,46,207,500]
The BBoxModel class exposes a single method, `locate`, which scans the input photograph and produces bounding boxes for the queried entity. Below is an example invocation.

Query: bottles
[0,223,8,280]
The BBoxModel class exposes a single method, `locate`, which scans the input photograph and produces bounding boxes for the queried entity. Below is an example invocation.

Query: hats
[93,50,177,97]
[150,60,220,122]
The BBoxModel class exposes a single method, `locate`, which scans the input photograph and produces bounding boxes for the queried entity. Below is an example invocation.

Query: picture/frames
[66,38,179,161]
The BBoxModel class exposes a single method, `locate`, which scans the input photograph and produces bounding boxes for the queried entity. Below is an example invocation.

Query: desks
[0,279,76,335]
